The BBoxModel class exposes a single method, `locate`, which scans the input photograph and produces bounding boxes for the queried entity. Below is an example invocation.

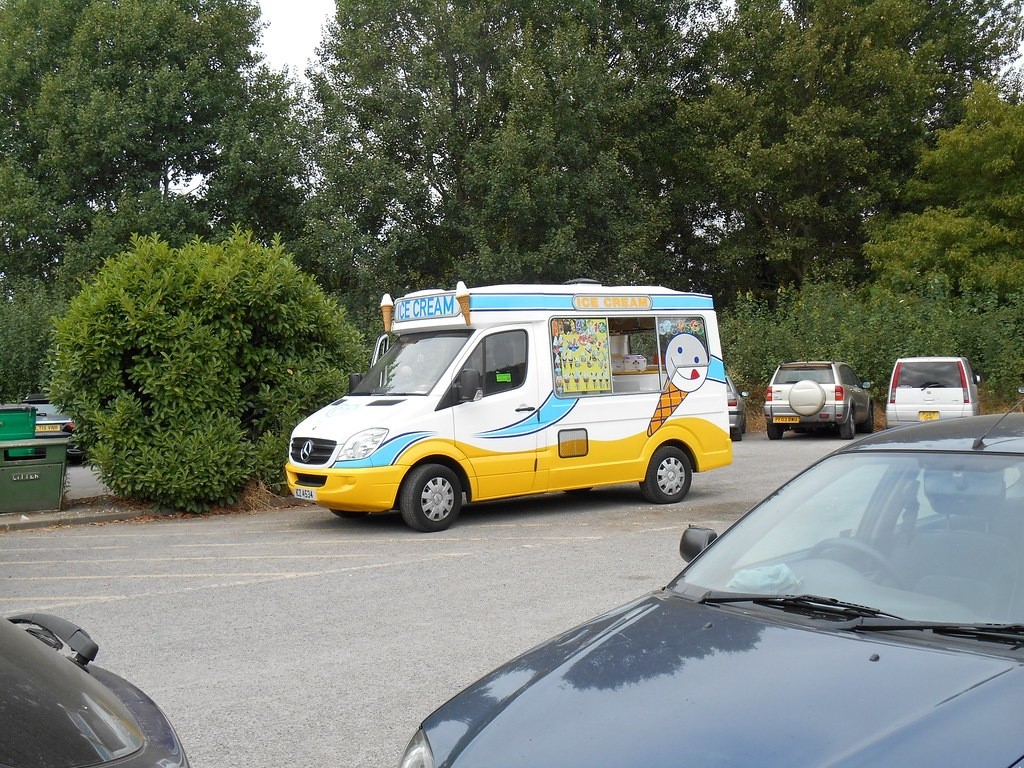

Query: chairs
[895,468,1020,620]
[492,344,520,386]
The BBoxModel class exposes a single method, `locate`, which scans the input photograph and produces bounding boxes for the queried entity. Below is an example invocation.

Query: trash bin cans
[0,404,38,456]
[0,439,71,514]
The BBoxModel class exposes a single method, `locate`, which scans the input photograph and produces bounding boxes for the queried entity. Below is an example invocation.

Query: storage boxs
[0,406,38,457]
[611,353,648,371]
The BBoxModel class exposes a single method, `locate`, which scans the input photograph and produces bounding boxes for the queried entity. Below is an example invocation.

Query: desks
[612,371,667,393]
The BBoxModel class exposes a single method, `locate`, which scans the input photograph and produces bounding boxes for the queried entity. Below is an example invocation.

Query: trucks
[282,274,735,529]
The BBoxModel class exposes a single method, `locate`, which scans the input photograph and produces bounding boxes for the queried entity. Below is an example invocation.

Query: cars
[398,411,1024,768]
[11,394,85,464]
[885,356,981,431]
[0,614,188,768]
[725,375,749,442]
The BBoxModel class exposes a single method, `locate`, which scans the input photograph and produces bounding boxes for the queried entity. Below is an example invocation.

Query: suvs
[764,360,874,442]
